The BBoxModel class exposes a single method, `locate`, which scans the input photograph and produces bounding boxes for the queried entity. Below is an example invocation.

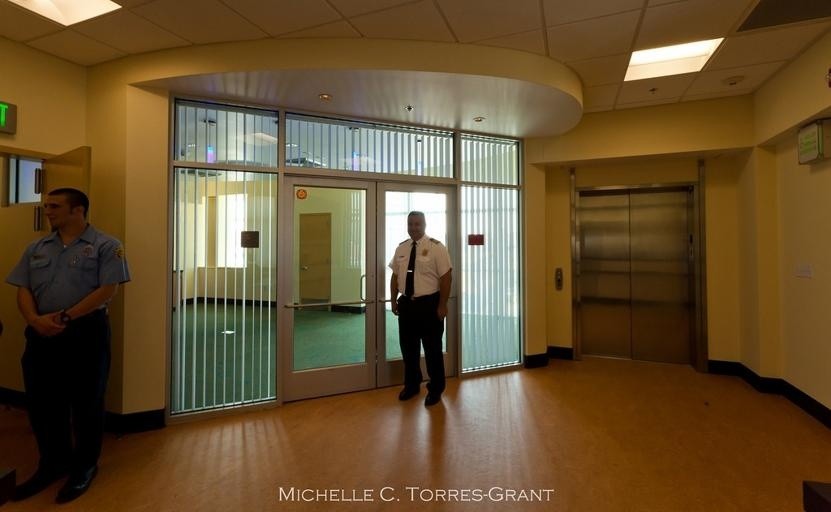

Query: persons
[5,189,131,502]
[388,209,453,405]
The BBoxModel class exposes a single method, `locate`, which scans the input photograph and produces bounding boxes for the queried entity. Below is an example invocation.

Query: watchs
[59,310,73,325]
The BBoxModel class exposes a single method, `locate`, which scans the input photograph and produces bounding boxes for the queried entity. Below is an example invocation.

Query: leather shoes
[398,385,421,401]
[425,391,441,407]
[14,465,57,495]
[57,472,92,503]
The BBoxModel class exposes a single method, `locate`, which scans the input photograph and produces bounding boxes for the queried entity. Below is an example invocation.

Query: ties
[404,240,417,299]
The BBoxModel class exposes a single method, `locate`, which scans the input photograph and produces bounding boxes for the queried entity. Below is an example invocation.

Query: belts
[402,293,431,301]
